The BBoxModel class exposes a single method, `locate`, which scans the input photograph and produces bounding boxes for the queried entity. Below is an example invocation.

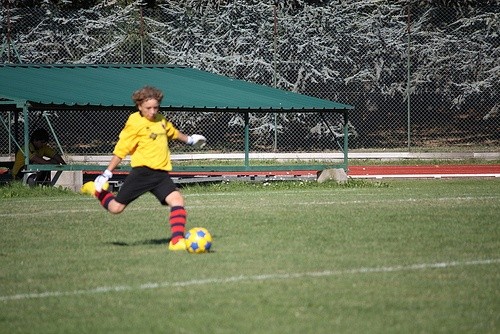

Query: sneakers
[80,181,109,196]
[167,238,186,250]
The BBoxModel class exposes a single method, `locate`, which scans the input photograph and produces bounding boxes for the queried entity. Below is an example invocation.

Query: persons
[80,87,207,251]
[12,128,65,185]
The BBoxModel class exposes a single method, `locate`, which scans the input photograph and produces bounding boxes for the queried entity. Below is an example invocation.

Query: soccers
[184,227,212,254]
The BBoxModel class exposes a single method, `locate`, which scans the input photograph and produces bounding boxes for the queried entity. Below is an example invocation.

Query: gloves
[186,134,206,150]
[94,169,113,193]
